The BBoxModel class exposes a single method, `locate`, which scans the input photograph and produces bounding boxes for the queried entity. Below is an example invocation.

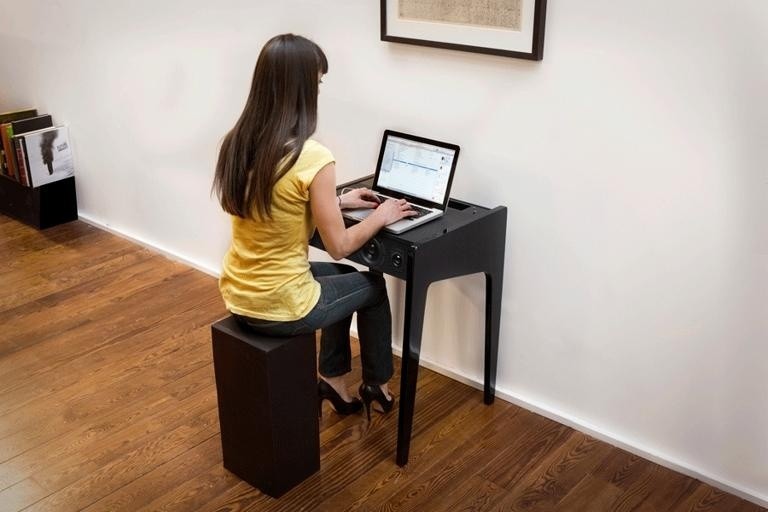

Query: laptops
[338,129,461,236]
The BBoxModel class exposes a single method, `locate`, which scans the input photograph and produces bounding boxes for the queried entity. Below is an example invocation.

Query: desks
[305,173,507,468]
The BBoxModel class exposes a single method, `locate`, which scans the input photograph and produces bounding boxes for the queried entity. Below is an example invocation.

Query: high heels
[359,382,394,422]
[317,377,364,418]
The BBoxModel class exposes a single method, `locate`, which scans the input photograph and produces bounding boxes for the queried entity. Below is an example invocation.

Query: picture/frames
[380,1,547,59]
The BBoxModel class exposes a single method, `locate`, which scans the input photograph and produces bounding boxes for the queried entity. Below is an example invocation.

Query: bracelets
[337,196,342,206]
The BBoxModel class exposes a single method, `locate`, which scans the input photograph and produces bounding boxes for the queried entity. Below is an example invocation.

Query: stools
[211,316,321,497]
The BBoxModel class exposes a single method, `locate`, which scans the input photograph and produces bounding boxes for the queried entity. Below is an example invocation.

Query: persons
[211,34,420,421]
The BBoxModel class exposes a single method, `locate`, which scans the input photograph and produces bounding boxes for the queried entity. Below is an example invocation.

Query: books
[1,109,75,189]
